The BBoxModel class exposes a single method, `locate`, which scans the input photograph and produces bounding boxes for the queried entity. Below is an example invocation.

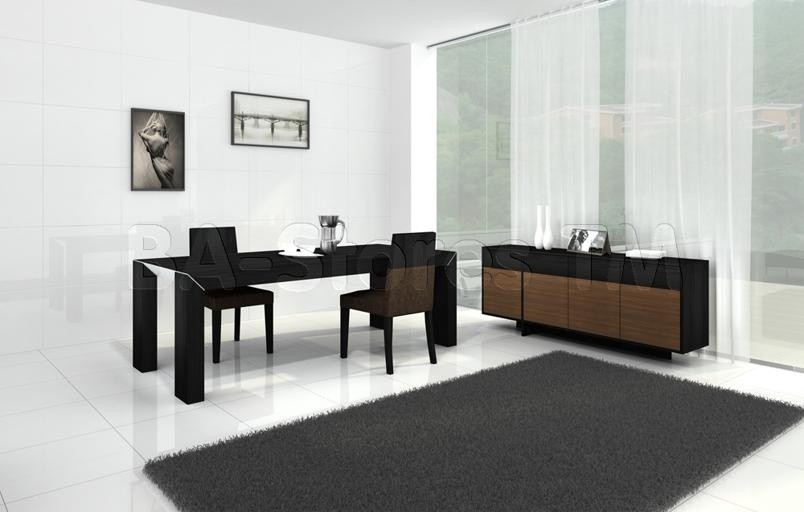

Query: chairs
[338,230,437,375]
[188,226,273,364]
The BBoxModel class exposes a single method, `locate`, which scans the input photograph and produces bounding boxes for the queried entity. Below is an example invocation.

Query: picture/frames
[129,108,185,192]
[230,91,309,150]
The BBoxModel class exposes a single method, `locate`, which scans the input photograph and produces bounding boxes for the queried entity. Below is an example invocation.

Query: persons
[567,229,589,251]
[136,119,175,188]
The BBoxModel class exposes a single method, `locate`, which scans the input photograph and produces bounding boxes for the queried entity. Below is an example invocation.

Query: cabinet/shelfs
[480,244,709,359]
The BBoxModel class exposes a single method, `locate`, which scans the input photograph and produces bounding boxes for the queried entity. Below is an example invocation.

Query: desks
[132,244,457,405]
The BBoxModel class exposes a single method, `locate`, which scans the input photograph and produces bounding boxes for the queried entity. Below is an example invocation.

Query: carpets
[140,349,804,511]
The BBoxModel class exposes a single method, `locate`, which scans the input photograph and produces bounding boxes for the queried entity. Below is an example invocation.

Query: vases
[533,205,553,251]
